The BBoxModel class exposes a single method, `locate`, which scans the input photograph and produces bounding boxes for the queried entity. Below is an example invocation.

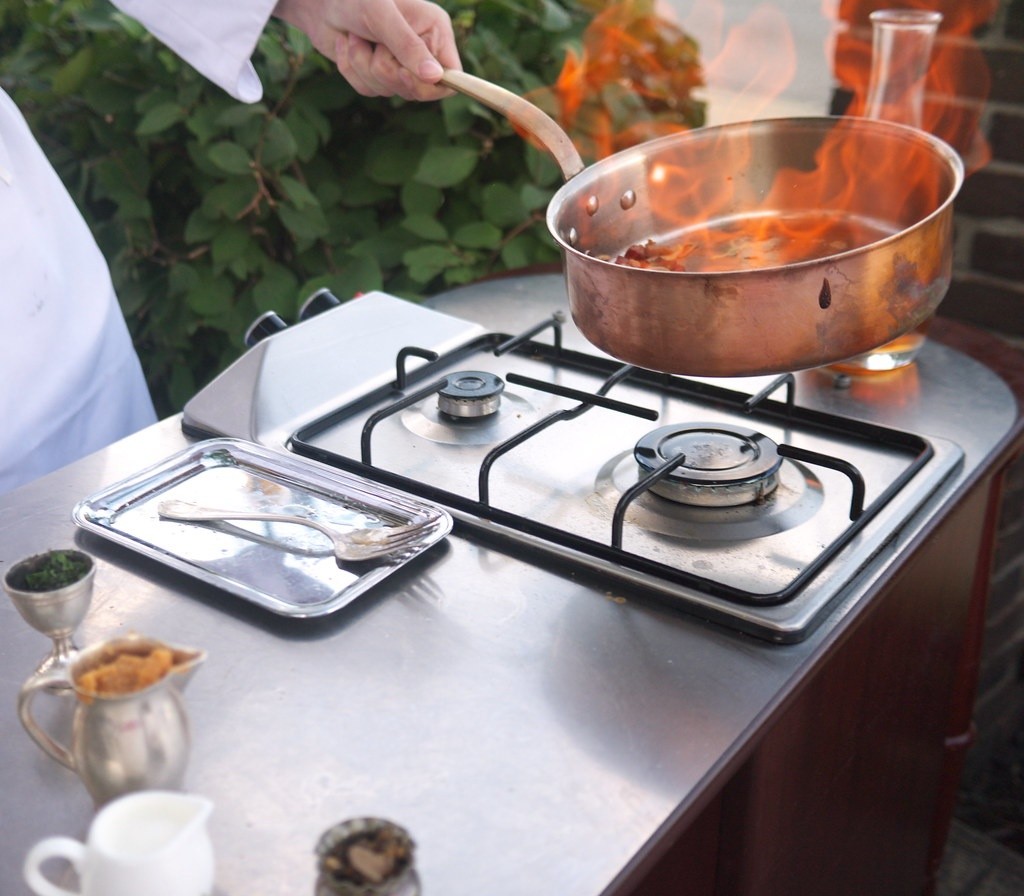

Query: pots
[440,67,967,377]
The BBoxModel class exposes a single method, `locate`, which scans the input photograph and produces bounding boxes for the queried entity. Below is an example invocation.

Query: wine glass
[1,548,97,697]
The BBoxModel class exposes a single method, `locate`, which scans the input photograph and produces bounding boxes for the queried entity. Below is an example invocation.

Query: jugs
[17,635,202,809]
[24,791,216,896]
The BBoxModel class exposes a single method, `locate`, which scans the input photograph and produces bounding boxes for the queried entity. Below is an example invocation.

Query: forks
[159,501,445,560]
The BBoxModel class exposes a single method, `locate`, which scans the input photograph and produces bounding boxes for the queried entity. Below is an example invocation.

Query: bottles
[817,8,946,371]
[314,818,421,896]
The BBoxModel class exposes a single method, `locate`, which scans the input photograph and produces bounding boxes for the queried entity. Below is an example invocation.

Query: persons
[0,2,465,496]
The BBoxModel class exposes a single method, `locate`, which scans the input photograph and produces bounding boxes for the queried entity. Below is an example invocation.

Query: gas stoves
[181,288,964,648]
